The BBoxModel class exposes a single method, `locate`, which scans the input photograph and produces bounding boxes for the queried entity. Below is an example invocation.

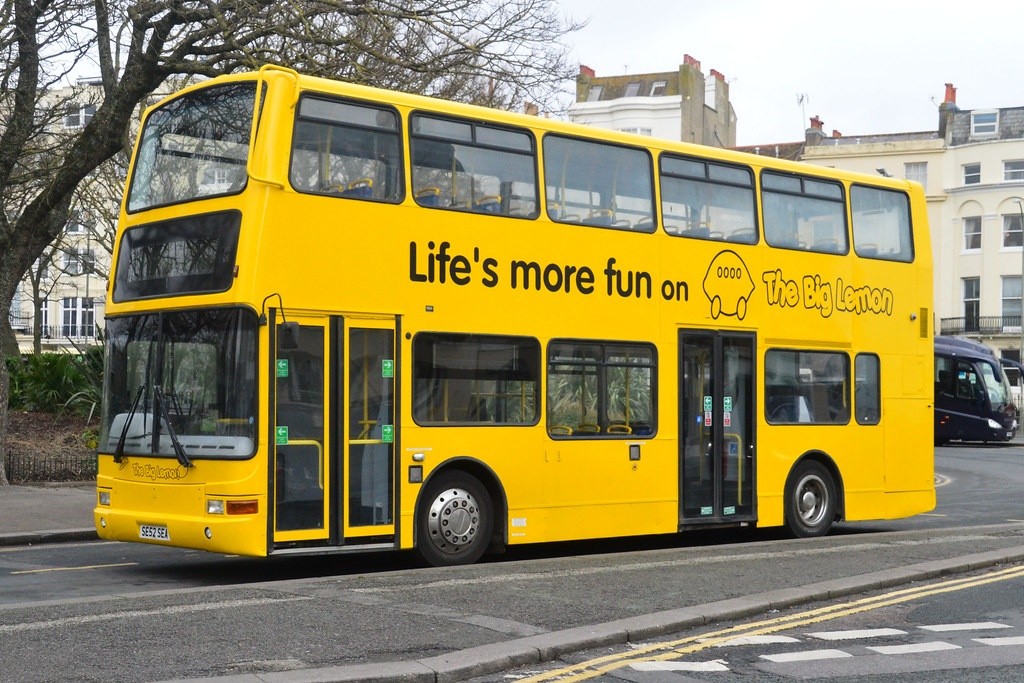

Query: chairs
[548,421,651,438]
[315,177,900,260]
[939,369,973,396]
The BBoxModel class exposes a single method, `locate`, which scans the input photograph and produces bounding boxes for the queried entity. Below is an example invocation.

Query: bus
[95,66,934,558]
[934,335,1024,445]
